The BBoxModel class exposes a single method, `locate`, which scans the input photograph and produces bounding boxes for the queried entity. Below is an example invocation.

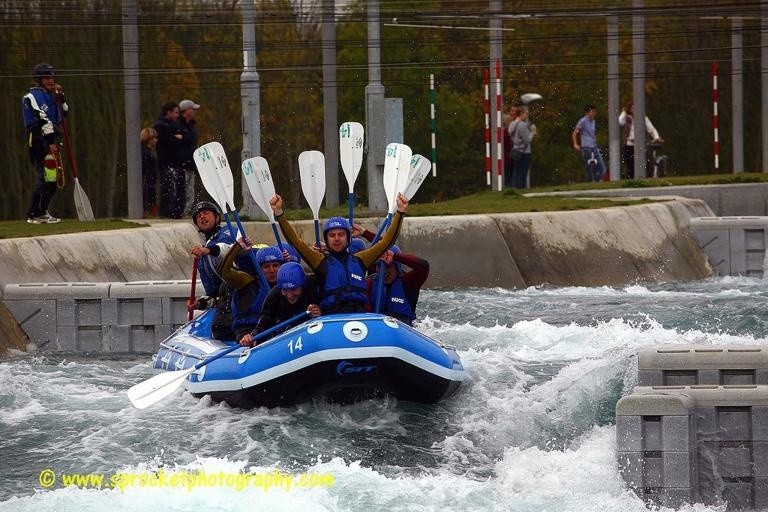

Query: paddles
[339,122,364,226]
[374,143,412,313]
[298,150,326,251]
[199,141,272,292]
[241,155,287,263]
[52,88,95,222]
[193,145,237,241]
[128,304,312,411]
[368,154,432,249]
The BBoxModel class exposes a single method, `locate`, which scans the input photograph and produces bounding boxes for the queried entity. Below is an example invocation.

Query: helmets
[33,63,55,82]
[256,243,307,289]
[192,201,221,224]
[376,245,401,272]
[323,217,366,253]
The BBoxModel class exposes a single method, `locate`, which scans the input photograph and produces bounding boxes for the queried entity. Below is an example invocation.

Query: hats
[179,99,200,111]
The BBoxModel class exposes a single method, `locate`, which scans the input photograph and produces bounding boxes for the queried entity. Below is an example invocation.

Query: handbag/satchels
[510,150,523,162]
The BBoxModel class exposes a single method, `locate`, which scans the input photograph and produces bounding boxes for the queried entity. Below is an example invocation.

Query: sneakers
[31,210,61,224]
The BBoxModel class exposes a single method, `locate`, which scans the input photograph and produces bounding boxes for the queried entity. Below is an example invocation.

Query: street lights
[520,91,545,190]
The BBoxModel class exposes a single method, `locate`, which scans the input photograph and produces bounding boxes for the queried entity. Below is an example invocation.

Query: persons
[140,128,160,218]
[503,104,537,189]
[178,99,201,218]
[619,103,663,179]
[186,193,430,347]
[22,63,69,225]
[572,104,607,182]
[152,102,186,220]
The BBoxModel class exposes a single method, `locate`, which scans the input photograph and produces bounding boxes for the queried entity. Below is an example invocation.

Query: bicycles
[621,137,668,180]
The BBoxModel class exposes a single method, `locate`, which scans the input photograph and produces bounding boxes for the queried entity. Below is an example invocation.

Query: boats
[151,303,465,414]
